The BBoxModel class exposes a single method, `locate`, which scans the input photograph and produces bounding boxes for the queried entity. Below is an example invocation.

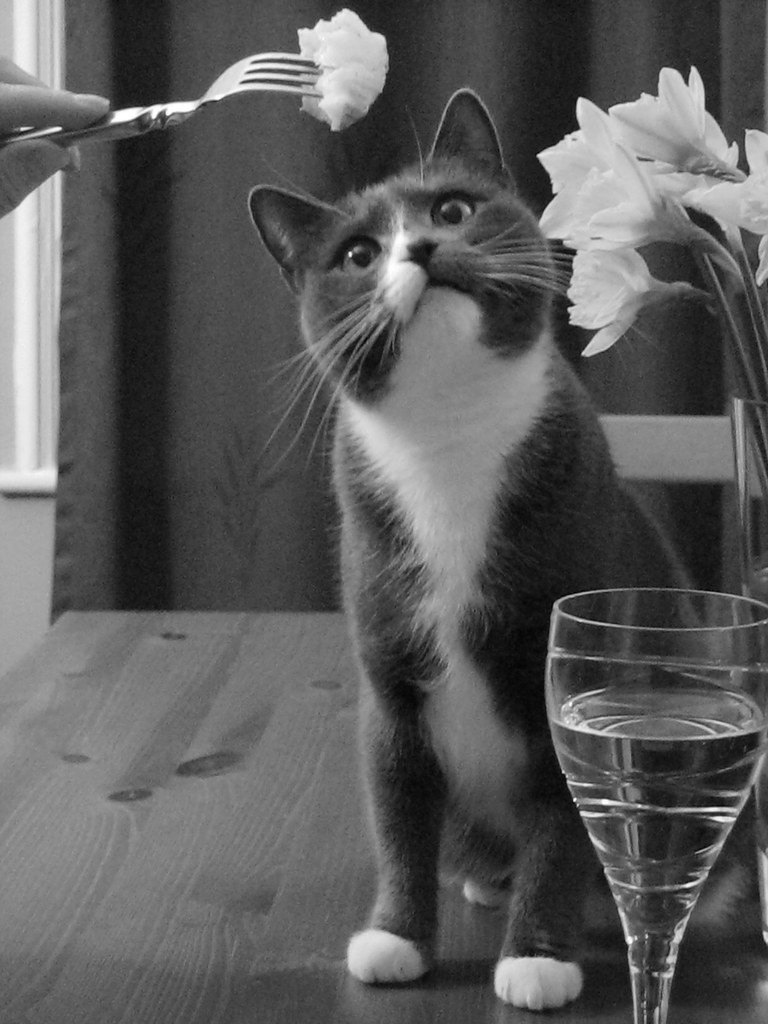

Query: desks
[2,609,767,1024]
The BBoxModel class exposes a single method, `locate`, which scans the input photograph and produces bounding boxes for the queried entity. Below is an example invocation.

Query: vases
[732,395,768,947]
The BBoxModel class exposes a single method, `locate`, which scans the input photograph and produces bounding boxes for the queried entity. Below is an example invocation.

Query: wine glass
[544,587,768,1024]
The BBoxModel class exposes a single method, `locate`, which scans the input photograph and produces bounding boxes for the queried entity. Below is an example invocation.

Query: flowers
[534,66,765,501]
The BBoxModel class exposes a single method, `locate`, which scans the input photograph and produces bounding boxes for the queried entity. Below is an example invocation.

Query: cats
[245,88,688,1011]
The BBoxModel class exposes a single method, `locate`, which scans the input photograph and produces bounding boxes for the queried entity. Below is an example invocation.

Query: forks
[0,52,326,148]
